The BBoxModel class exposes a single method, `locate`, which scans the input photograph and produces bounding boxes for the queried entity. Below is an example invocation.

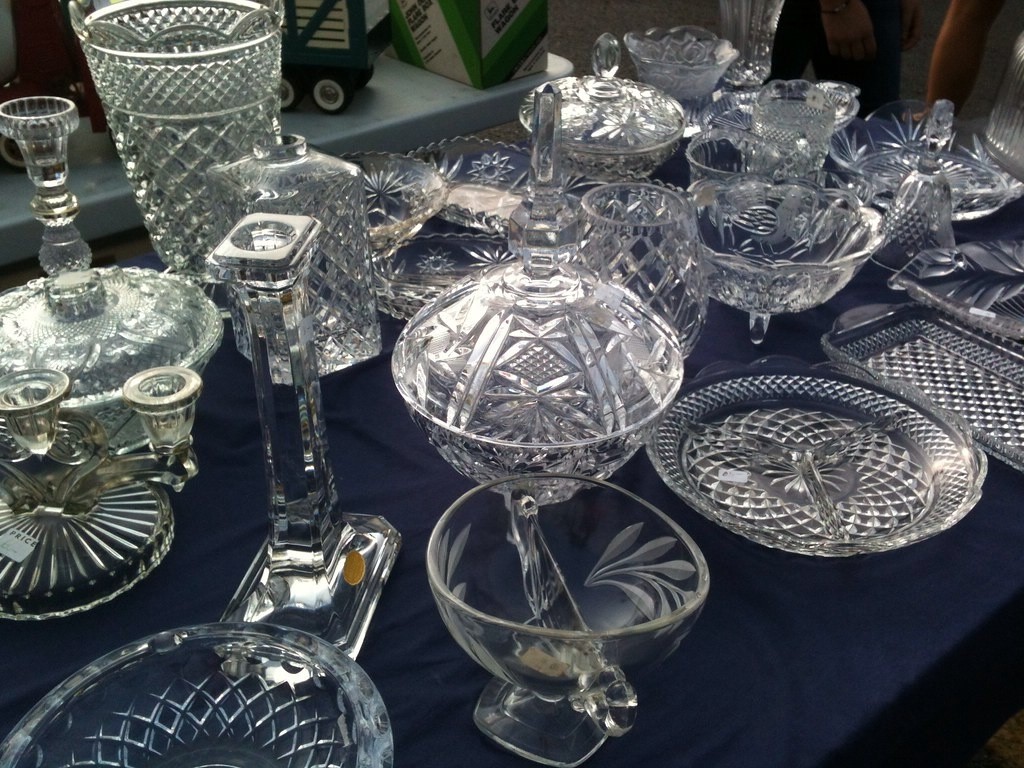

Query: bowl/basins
[519,34,681,180]
[0,620,395,768]
[687,135,782,190]
[0,268,225,462]
[406,137,537,229]
[373,232,522,324]
[342,148,447,258]
[685,175,877,317]
[390,264,683,502]
[622,26,738,137]
[829,109,1024,220]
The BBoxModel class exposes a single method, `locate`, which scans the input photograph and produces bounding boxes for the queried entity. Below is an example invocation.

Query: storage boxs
[384,0,548,90]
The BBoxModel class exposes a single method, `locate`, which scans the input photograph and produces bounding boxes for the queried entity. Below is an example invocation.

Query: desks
[0,52,573,266]
[0,80,1023,768]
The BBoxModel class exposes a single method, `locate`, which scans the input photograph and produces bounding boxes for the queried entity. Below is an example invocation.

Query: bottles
[870,99,958,277]
[721,0,785,87]
[208,131,383,377]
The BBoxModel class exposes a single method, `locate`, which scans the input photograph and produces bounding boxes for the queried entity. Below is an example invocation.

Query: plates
[699,87,860,136]
[642,353,987,556]
[822,303,1024,472]
[887,237,1024,334]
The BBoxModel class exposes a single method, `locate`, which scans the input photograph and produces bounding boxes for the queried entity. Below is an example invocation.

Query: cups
[579,180,707,360]
[65,0,286,308]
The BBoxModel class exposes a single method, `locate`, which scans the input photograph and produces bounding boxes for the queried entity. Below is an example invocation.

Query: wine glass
[425,472,711,768]
